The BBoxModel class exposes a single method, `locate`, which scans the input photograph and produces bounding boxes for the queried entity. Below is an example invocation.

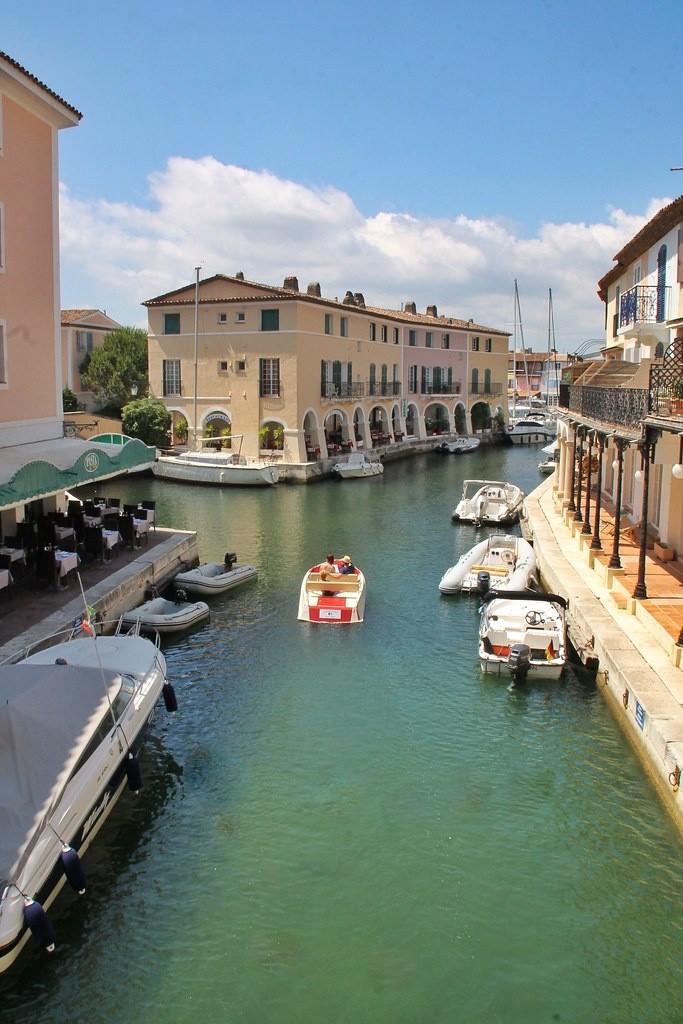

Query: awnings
[0,438,161,512]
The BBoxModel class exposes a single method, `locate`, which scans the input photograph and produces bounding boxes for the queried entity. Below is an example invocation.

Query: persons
[339,556,354,574]
[319,554,335,596]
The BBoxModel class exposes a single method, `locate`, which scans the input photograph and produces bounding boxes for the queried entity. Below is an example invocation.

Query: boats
[173,551,257,594]
[477,592,569,687]
[539,457,555,474]
[438,437,481,454]
[114,589,212,633]
[334,454,384,479]
[0,615,179,992]
[437,532,538,595]
[297,559,367,625]
[452,479,525,526]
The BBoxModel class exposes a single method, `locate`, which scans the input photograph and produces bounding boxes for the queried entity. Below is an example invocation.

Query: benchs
[470,562,510,577]
[523,629,561,652]
[305,573,360,599]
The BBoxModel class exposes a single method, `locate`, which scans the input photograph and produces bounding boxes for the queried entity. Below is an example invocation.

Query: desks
[34,524,76,552]
[94,504,119,521]
[133,518,148,550]
[83,515,101,528]
[102,530,123,561]
[137,508,156,534]
[0,569,16,604]
[0,548,27,581]
[54,551,82,588]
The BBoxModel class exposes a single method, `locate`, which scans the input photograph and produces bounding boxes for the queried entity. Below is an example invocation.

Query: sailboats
[150,266,283,485]
[508,279,557,444]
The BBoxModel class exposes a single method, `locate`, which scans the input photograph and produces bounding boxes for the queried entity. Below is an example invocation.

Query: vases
[654,542,674,562]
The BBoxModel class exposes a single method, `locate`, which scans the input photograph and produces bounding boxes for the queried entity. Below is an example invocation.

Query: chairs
[0,497,156,594]
[306,430,405,461]
[486,631,511,647]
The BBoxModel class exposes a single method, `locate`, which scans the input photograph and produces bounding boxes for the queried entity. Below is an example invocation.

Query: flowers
[582,455,600,474]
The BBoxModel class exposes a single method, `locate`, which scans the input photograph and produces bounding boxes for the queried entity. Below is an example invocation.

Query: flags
[545,640,554,660]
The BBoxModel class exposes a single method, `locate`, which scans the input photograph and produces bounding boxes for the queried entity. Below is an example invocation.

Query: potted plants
[675,378,683,415]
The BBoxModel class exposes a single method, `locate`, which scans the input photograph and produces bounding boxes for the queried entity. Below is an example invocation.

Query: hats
[339,555,351,563]
